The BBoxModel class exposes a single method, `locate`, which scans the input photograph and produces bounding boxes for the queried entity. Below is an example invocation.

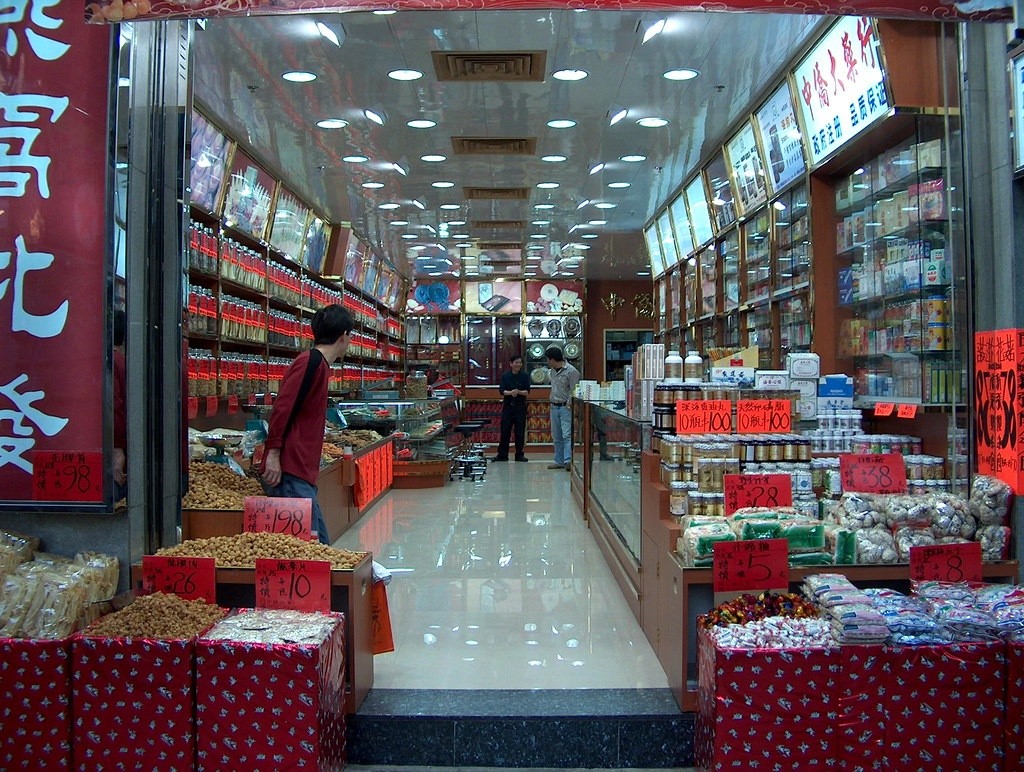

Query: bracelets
[567,406,571,409]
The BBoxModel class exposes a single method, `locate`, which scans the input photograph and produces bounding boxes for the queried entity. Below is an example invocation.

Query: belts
[508,401,520,406]
[551,402,567,406]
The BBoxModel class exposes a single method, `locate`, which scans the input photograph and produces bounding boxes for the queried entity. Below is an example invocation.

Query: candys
[696,588,837,651]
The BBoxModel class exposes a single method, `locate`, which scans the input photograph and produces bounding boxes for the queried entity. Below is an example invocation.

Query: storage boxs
[0,633,73,772]
[194,606,349,772]
[696,614,1024,772]
[73,605,230,772]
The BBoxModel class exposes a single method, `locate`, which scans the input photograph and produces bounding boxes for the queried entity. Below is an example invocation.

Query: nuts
[77,462,364,642]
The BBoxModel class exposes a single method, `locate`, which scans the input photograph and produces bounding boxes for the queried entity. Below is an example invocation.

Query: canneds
[650,383,951,516]
[946,429,969,498]
[877,295,946,350]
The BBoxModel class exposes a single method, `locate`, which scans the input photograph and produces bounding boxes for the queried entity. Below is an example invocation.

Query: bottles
[664,351,683,384]
[685,351,702,383]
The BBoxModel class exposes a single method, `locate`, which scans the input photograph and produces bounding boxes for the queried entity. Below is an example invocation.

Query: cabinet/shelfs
[570,395,622,521]
[641,448,1018,713]
[131,552,375,715]
[184,507,245,541]
[188,266,406,431]
[406,335,582,442]
[653,104,965,413]
[584,401,655,626]
[315,435,394,545]
[336,398,461,489]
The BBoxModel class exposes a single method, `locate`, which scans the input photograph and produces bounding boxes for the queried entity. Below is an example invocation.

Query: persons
[545,347,580,470]
[259,303,354,543]
[492,355,530,462]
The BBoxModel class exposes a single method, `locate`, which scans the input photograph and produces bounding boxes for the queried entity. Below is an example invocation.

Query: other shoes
[547,464,565,469]
[566,464,571,472]
[491,453,509,461]
[515,452,528,462]
[600,453,612,460]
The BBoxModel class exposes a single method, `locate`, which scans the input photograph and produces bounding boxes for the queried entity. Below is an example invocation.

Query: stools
[448,417,493,481]
